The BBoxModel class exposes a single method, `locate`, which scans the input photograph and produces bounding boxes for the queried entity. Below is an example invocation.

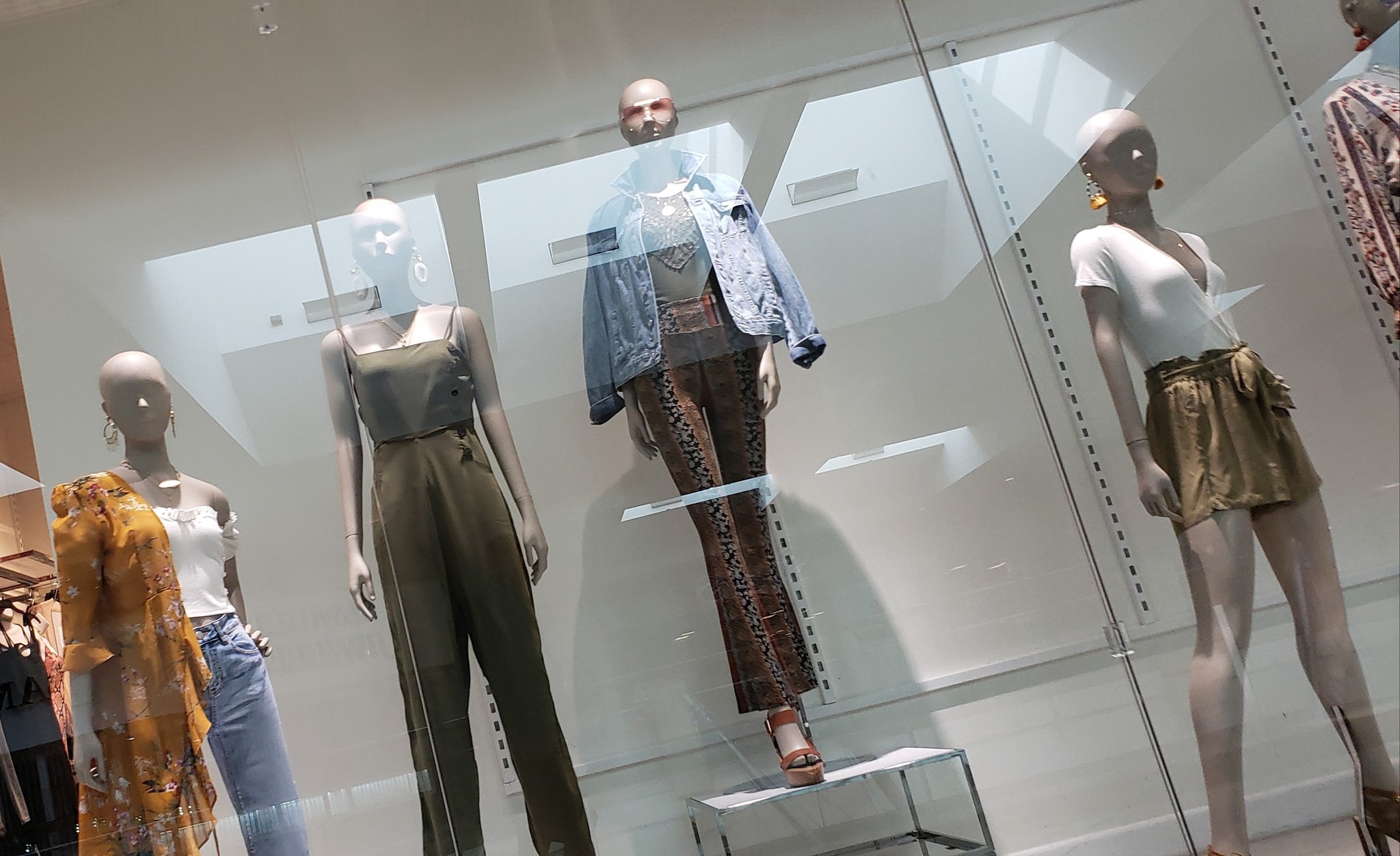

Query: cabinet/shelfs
[681,747,1000,856]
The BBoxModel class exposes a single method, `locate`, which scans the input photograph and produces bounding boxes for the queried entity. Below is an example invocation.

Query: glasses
[618,98,679,131]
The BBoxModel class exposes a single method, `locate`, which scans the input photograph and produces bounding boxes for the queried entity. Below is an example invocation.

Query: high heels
[1363,787,1400,856]
[766,709,824,785]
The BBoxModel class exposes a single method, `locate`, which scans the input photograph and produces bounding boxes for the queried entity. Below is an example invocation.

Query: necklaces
[368,302,419,347]
[120,459,181,503]
[1366,60,1399,80]
[1107,208,1153,218]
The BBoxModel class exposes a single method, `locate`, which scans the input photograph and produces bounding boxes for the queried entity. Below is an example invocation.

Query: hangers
[0,592,42,625]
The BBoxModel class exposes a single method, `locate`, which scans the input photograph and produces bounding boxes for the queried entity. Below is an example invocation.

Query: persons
[320,200,599,856]
[1070,110,1399,856]
[0,606,80,856]
[1321,0,1400,341]
[581,79,827,786]
[49,351,311,856]
